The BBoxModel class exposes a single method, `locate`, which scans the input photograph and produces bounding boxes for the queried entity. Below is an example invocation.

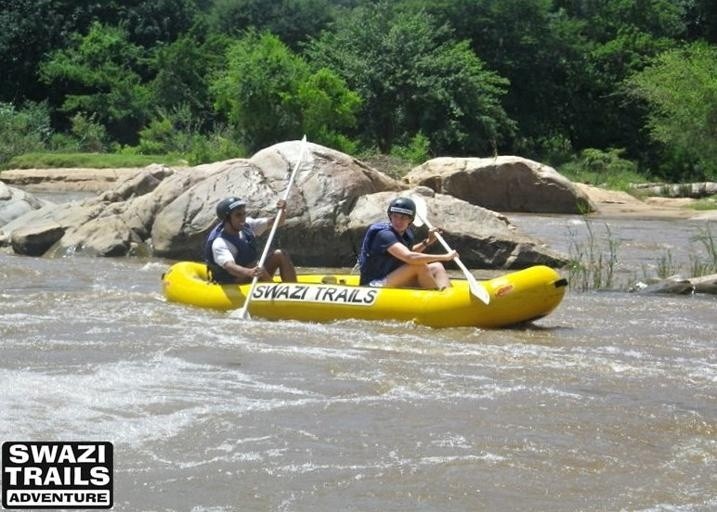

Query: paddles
[409,195,490,305]
[228,135,307,320]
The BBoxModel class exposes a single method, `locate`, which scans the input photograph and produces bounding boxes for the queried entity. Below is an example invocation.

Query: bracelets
[422,239,428,246]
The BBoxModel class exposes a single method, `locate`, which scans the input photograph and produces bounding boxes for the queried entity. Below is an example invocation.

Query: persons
[356,196,459,290]
[202,196,297,283]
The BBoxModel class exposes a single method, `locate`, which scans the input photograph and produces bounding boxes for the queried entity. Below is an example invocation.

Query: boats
[166,259,566,329]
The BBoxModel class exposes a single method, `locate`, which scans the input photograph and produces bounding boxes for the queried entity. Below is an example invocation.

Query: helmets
[216,196,246,221]
[387,197,416,221]
